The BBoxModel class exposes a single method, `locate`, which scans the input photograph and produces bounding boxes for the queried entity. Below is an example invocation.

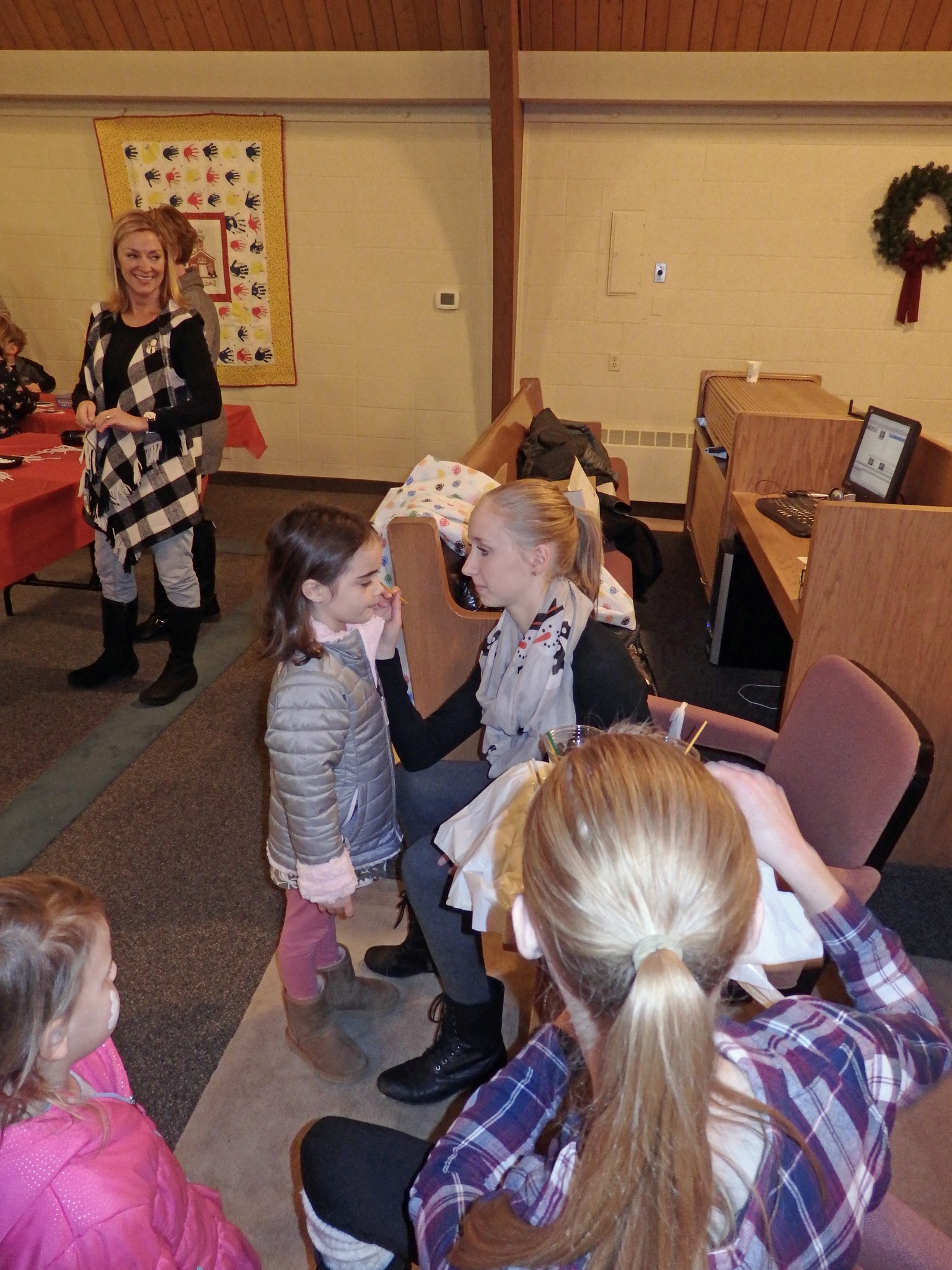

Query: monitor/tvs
[841,404,921,504]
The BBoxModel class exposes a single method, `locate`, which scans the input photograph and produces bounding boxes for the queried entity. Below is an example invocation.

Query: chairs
[647,654,935,996]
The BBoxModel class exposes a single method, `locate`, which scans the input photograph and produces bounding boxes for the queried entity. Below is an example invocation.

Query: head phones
[829,489,856,502]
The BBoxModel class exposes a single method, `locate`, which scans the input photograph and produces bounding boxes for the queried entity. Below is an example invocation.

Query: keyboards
[755,497,819,538]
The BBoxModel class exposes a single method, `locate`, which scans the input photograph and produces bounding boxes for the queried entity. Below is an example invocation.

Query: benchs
[386,377,633,761]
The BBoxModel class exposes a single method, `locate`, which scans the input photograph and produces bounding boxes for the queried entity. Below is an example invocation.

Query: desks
[721,412,952,870]
[19,394,268,460]
[0,433,103,619]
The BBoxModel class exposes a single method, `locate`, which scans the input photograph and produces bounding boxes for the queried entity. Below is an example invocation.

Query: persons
[301,731,952,1270]
[65,208,222,710]
[1,875,266,1270]
[0,301,37,441]
[364,477,654,1106]
[5,323,56,403]
[134,204,222,644]
[257,502,403,1084]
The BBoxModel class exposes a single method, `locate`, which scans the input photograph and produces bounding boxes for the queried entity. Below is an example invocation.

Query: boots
[281,973,367,1084]
[315,938,402,1016]
[376,973,509,1102]
[363,890,442,981]
[135,557,173,642]
[190,519,221,622]
[68,594,142,690]
[139,607,198,705]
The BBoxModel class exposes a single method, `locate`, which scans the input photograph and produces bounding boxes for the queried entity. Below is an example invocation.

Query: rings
[106,413,110,420]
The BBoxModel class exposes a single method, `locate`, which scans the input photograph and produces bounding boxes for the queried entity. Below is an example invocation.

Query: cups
[659,735,701,762]
[544,724,607,764]
[746,361,761,383]
